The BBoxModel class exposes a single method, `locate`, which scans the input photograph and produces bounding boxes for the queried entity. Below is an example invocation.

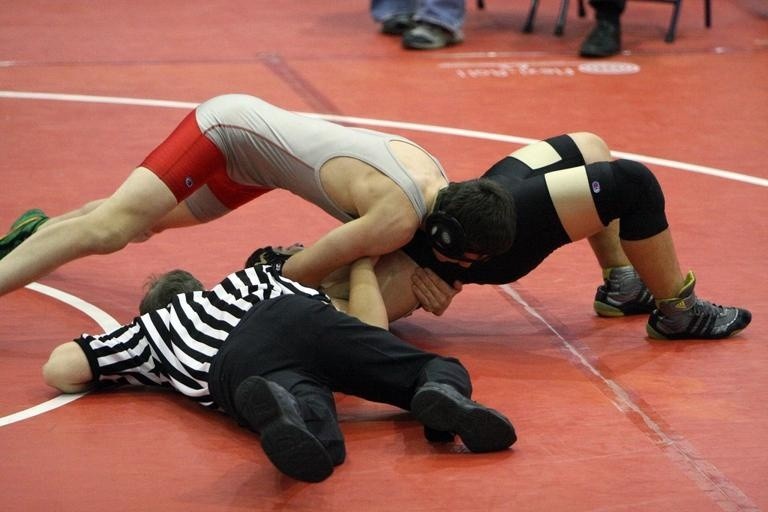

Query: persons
[243,120,753,351]
[40,251,519,487]
[370,0,474,51]
[1,86,516,333]
[575,0,637,59]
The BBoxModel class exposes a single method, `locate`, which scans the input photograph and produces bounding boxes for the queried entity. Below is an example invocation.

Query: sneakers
[580,21,624,58]
[645,269,753,343]
[402,22,455,51]
[0,207,51,261]
[409,381,519,454]
[592,264,660,318]
[234,374,336,484]
[383,16,412,36]
